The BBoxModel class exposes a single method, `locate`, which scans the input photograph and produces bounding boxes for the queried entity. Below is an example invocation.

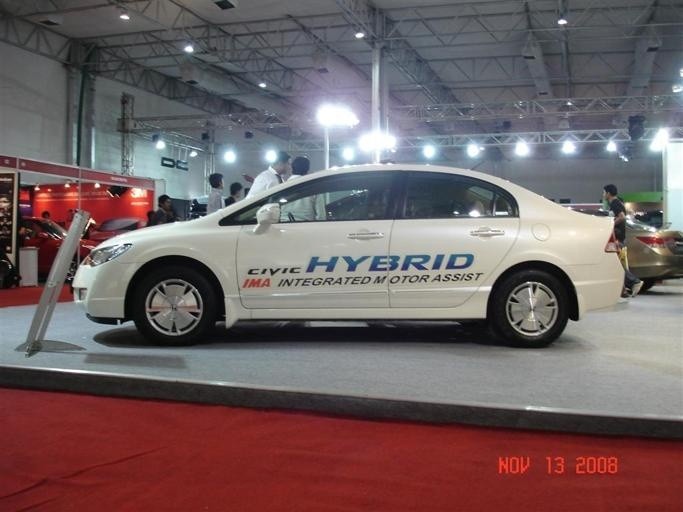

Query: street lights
[317,105,359,169]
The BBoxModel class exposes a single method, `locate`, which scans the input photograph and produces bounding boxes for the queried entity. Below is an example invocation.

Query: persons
[277,154,329,222]
[151,194,173,226]
[601,184,645,299]
[205,173,225,215]
[225,182,241,207]
[243,151,292,225]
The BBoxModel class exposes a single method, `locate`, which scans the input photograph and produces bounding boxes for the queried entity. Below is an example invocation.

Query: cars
[560,202,683,293]
[90,217,138,242]
[67,163,627,347]
[17,213,101,284]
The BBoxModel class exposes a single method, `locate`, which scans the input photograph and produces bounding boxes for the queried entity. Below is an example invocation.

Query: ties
[275,174,283,184]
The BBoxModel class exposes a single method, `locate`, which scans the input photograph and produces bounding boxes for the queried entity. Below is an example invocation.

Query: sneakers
[631,280,644,297]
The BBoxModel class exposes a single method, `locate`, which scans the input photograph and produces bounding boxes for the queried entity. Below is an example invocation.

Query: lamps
[672,83,681,93]
[650,128,670,152]
[562,141,575,153]
[224,152,235,162]
[161,156,188,169]
[467,145,480,156]
[317,106,358,127]
[119,10,131,20]
[245,132,253,138]
[355,30,366,39]
[184,45,194,53]
[628,115,644,140]
[557,17,568,25]
[424,145,437,156]
[258,81,267,88]
[266,151,277,162]
[515,144,530,156]
[342,149,353,159]
[201,132,209,140]
[190,151,197,157]
[607,143,617,152]
[360,134,396,150]
[152,135,166,149]
